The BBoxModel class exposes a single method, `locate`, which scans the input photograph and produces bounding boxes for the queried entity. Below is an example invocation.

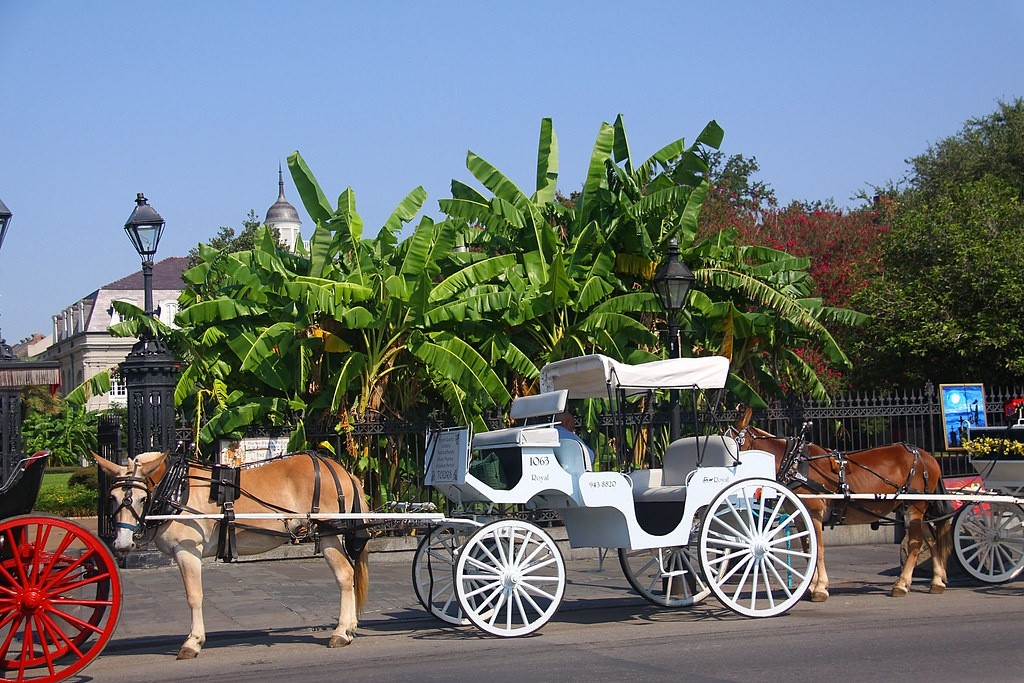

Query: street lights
[648,237,700,598]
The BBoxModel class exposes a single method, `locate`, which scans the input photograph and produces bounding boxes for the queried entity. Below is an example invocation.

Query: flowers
[962,438,1024,458]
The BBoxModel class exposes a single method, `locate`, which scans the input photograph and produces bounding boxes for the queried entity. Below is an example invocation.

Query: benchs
[473,389,568,448]
[0,450,51,559]
[555,439,633,491]
[627,436,739,502]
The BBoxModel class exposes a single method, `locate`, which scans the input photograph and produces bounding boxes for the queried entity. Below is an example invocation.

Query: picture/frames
[939,383,988,451]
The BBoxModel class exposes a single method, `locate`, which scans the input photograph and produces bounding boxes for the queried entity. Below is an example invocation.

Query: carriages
[721,404,1024,605]
[86,351,821,661]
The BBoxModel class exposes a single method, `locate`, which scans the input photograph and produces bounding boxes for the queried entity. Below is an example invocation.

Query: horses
[90,449,372,660]
[732,406,950,602]
